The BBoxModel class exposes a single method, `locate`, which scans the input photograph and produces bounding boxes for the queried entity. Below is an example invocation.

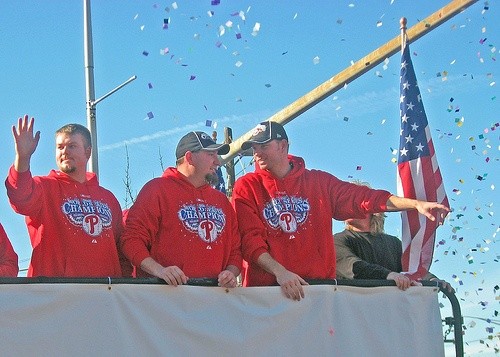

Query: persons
[332,179,452,293]
[232,120,453,301]
[116,209,156,279]
[119,131,242,288]
[5,114,156,278]
[0,223,19,278]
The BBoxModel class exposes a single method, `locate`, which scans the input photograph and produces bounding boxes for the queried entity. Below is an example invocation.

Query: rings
[232,279,234,282]
[229,276,232,279]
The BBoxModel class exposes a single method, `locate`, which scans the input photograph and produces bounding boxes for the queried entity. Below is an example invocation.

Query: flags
[397,34,451,286]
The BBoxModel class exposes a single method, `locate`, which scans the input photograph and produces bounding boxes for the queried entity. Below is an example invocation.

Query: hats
[241,121,287,151]
[176,132,230,161]
